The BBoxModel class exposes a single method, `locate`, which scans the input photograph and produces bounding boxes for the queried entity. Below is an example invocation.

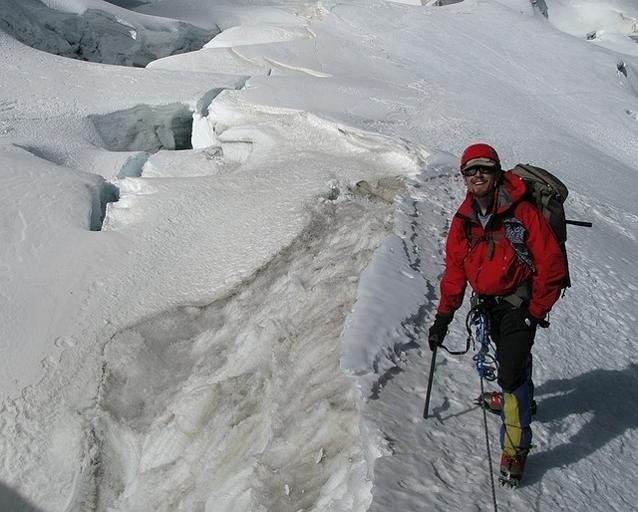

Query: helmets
[462,144,500,167]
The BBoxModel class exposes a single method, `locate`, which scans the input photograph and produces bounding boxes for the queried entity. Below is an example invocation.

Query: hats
[464,159,495,170]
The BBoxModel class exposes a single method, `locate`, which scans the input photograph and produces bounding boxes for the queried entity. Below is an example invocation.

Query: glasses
[462,165,492,175]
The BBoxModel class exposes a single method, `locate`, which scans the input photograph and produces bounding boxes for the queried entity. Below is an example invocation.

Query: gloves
[531,316,549,329]
[428,317,448,348]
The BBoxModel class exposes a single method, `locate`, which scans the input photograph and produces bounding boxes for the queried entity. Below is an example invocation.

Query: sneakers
[499,453,524,479]
[480,391,536,415]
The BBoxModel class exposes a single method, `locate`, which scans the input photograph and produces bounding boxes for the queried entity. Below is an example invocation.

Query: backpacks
[503,163,571,286]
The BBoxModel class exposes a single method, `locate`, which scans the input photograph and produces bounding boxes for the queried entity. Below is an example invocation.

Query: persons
[430,143,571,490]
